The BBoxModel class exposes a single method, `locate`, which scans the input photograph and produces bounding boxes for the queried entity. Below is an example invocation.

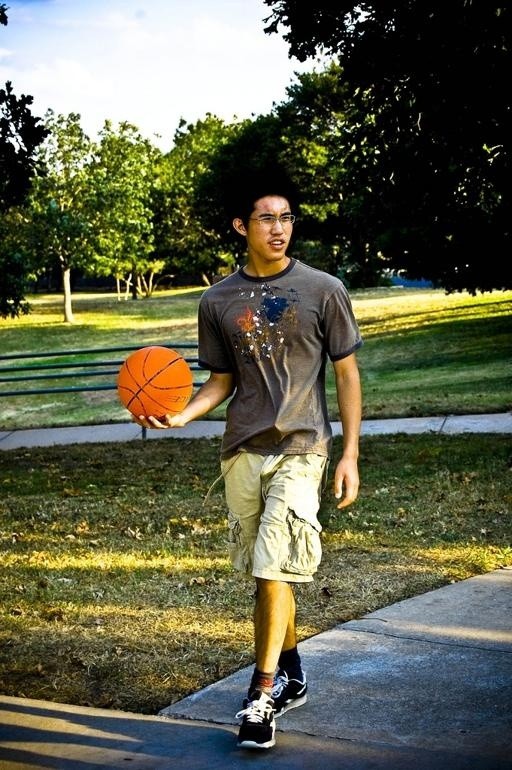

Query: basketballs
[117,346,192,422]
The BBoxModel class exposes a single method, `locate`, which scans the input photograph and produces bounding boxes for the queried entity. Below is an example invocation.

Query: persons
[130,188,364,749]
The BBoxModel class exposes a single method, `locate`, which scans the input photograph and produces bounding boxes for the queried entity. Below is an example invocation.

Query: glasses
[249,215,295,227]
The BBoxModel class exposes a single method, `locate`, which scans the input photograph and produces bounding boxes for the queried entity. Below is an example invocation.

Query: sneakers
[272,669,308,718]
[236,688,277,749]
